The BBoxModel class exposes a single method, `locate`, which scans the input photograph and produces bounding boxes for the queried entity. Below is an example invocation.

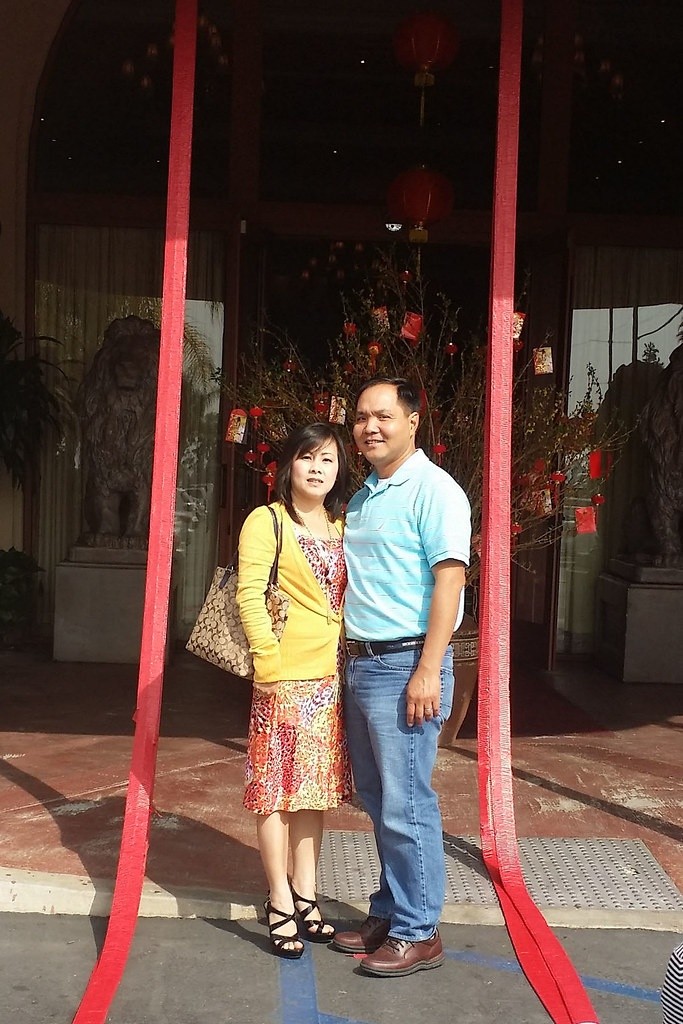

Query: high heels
[263,891,305,958]
[291,885,336,942]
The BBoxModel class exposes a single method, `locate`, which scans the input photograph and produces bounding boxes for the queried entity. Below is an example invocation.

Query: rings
[425,708,432,711]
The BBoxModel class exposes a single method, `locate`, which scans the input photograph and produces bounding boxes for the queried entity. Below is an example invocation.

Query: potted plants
[230,246,638,743]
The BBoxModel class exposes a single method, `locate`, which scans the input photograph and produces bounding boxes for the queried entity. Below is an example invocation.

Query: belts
[345,635,426,656]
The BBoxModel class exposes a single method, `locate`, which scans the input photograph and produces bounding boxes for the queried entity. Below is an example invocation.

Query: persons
[330,378,472,976]
[235,424,353,958]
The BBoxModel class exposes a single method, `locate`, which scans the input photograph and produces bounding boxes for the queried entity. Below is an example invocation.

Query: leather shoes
[333,917,392,953]
[360,928,445,976]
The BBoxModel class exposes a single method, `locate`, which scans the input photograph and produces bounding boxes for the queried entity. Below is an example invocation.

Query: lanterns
[390,6,457,124]
[383,162,456,284]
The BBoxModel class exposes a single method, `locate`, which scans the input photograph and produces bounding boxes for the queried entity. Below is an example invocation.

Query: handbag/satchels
[185,507,291,681]
